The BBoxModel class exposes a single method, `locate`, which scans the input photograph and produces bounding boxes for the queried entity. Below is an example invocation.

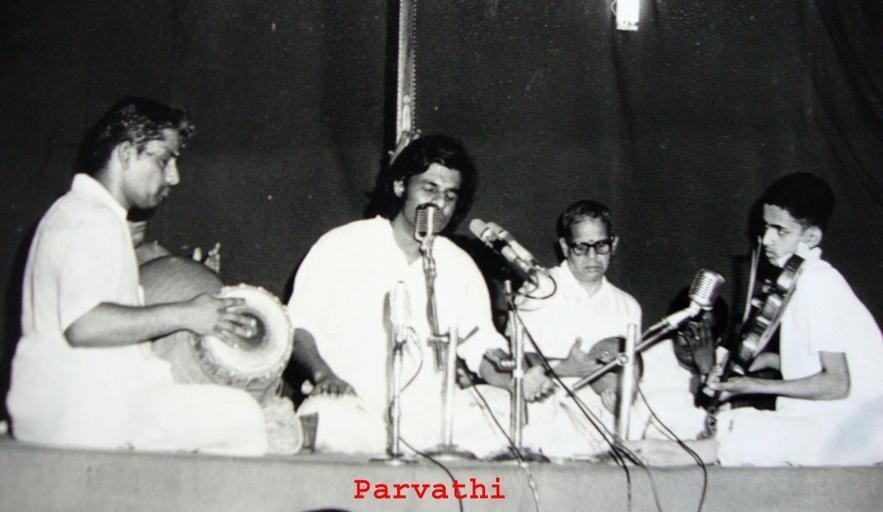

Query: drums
[139,255,294,404]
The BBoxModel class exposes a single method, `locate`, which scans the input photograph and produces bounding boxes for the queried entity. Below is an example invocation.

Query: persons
[504,201,643,465]
[630,173,882,465]
[7,99,270,453]
[287,135,552,458]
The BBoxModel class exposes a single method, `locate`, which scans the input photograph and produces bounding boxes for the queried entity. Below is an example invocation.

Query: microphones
[388,288,411,342]
[665,267,726,329]
[469,218,532,274]
[414,201,443,257]
[485,221,550,277]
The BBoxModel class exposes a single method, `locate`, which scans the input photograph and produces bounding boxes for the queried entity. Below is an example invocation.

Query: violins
[704,255,804,434]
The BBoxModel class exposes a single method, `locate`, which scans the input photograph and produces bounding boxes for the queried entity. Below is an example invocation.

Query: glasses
[565,235,615,256]
